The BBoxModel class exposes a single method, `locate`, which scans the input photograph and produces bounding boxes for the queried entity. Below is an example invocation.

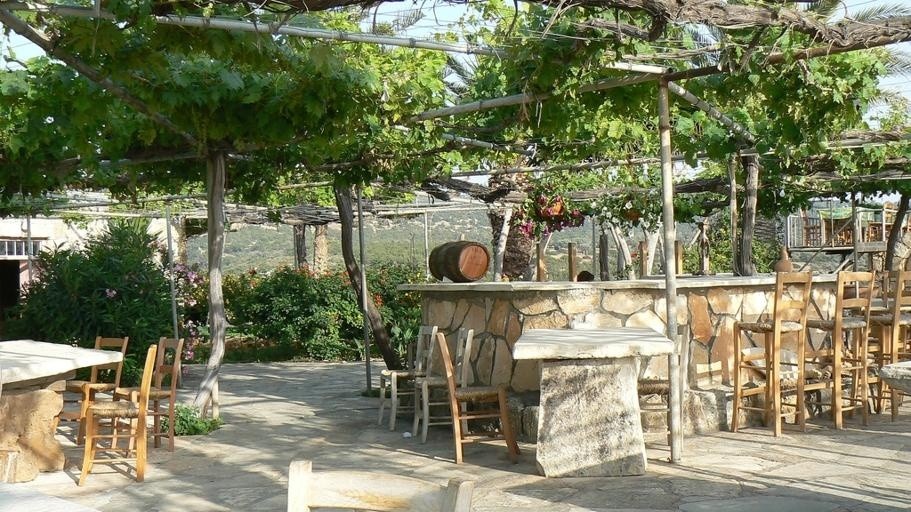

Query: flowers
[495,178,586,238]
[586,190,664,238]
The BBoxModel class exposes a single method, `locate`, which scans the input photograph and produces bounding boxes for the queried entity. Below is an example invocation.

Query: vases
[540,202,563,216]
[624,209,641,221]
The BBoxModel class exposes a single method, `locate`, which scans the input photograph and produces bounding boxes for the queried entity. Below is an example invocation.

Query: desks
[877,360,911,396]
[0,339,123,482]
[511,327,674,477]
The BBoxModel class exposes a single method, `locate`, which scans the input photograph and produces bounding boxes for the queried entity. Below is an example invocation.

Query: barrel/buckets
[428,240,490,283]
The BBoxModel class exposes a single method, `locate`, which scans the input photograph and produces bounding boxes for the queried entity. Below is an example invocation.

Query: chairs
[77,344,158,487]
[796,269,876,430]
[866,267,911,423]
[287,459,475,512]
[377,325,439,430]
[412,327,474,444]
[56,336,129,446]
[112,336,185,459]
[437,331,521,465]
[729,267,813,438]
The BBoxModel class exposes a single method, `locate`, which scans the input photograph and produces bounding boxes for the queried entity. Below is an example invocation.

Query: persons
[576,270,595,281]
